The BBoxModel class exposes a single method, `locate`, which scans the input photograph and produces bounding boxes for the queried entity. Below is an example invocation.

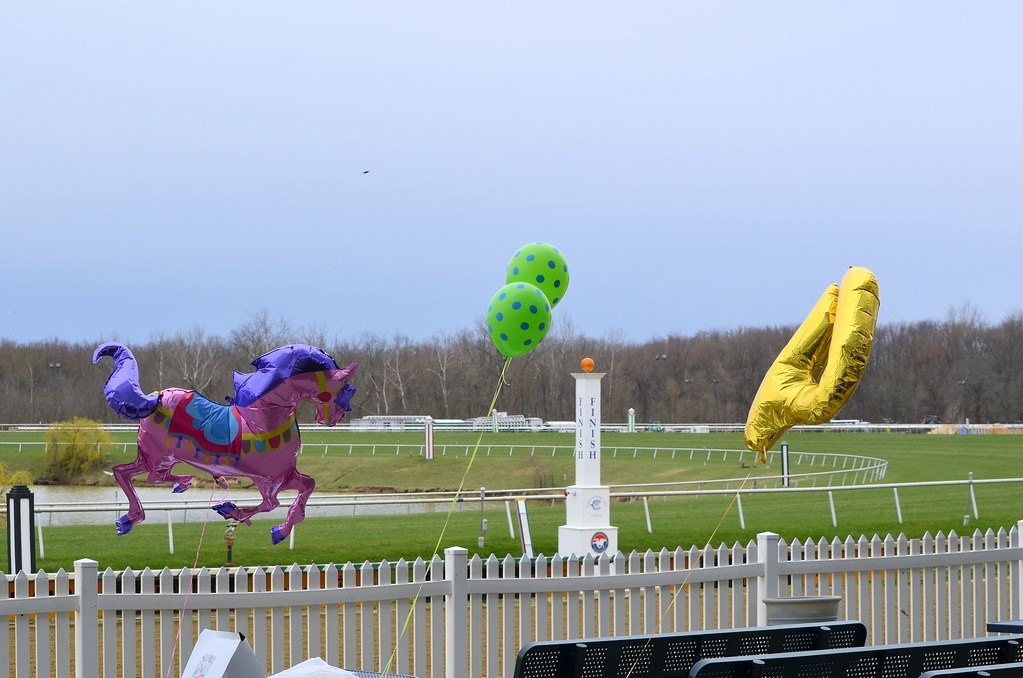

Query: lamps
[5,483,36,574]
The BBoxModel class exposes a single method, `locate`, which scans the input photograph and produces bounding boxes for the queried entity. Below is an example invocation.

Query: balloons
[487,282,553,360]
[92,341,359,545]
[506,242,570,309]
[745,266,880,465]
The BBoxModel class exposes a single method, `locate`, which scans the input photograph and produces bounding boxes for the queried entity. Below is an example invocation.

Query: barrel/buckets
[761,595,843,626]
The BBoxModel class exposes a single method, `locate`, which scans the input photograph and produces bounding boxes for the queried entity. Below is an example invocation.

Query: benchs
[513,619,867,678]
[689,634,1022,677]
